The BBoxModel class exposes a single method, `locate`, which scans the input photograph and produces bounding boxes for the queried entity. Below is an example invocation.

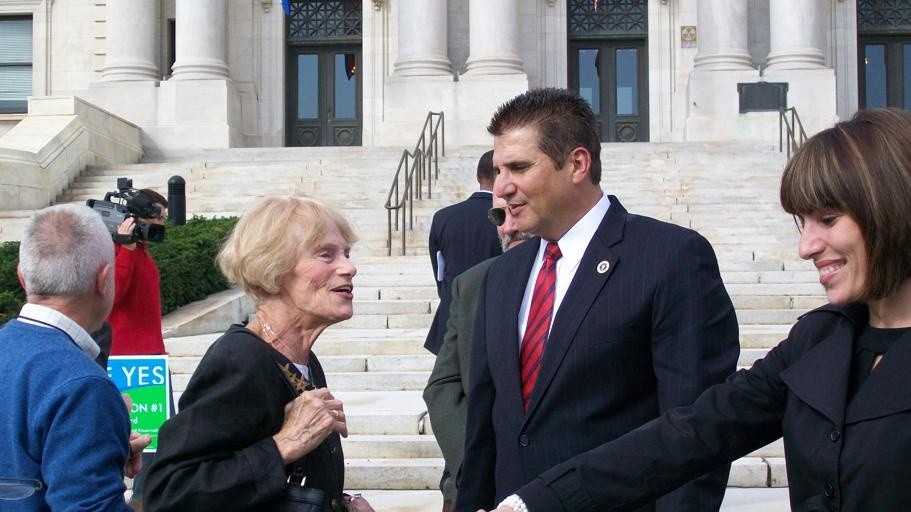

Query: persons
[105,189,175,417]
[0,203,152,510]
[137,199,376,511]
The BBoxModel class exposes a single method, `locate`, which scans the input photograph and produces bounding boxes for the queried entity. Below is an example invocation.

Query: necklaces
[254,309,318,389]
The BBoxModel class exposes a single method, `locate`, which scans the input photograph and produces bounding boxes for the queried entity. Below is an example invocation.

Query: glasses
[487,205,510,226]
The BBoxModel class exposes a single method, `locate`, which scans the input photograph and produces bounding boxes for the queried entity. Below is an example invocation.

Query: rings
[332,409,340,420]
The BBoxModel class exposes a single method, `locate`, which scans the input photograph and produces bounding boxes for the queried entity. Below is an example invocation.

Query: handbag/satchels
[274,484,326,512]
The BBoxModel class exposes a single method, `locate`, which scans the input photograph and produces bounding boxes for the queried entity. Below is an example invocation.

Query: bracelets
[345,493,362,509]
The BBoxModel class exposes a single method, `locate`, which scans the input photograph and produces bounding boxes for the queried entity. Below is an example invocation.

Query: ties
[521,241,562,416]
